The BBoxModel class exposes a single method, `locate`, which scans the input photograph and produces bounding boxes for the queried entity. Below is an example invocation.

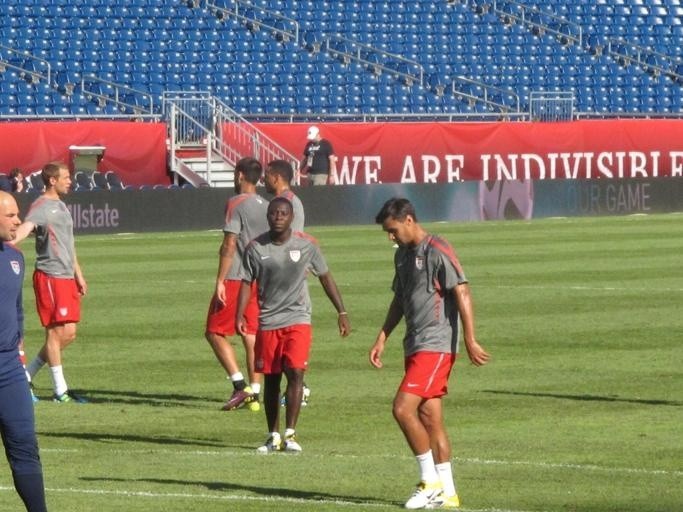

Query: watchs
[337,312,347,314]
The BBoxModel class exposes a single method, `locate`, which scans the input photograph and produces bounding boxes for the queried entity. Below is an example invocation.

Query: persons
[300,125,336,185]
[234,198,350,454]
[368,195,490,510]
[9,168,28,192]
[262,160,311,406]
[1,241,38,402]
[8,162,87,403]
[204,156,271,411]
[0,188,46,511]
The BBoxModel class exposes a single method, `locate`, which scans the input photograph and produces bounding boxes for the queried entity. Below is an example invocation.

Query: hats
[306,126,319,140]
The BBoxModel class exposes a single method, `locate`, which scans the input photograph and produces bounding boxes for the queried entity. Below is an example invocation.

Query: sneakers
[221,387,259,413]
[256,432,302,452]
[52,389,89,405]
[404,476,459,508]
[279,383,309,406]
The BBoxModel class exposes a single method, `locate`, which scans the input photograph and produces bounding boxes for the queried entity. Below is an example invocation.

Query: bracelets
[330,174,333,176]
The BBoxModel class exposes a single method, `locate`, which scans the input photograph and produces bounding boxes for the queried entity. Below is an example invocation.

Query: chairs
[0,167,196,190]
[2,0,683,120]
